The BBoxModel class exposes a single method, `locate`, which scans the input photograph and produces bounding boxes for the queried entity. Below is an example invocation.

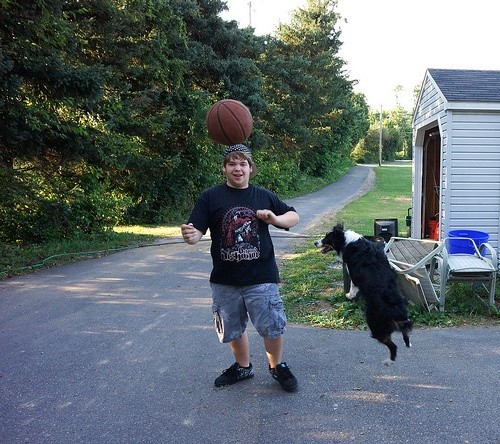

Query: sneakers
[214,362,254,387]
[268,362,298,391]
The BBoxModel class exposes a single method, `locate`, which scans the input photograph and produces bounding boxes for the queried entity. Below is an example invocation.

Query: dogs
[313,222,413,361]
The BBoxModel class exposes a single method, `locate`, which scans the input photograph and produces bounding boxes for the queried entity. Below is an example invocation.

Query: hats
[223,145,258,178]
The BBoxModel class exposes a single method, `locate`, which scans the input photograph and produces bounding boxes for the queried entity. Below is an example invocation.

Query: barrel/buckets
[450,228,489,259]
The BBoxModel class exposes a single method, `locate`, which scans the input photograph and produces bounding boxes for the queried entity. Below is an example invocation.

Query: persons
[180,143,300,392]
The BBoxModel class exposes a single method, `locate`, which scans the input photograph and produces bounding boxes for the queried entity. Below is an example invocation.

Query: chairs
[381,233,499,312]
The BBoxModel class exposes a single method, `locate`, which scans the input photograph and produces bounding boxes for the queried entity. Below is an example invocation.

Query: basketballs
[206,98,252,144]
